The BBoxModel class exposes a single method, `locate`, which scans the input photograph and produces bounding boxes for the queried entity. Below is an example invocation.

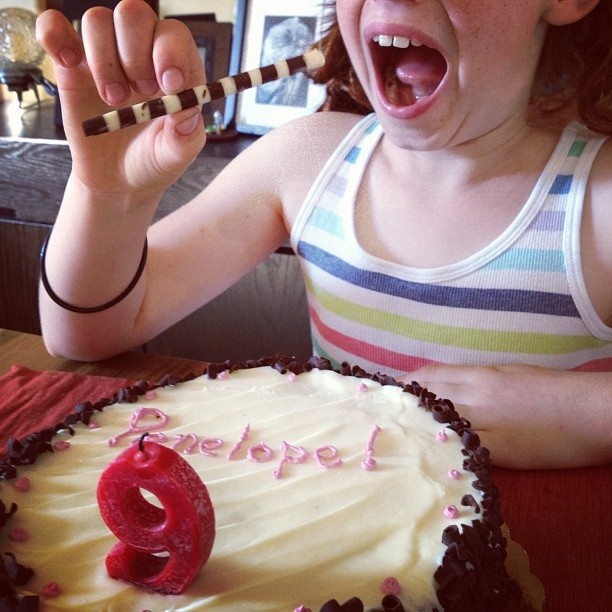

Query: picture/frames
[160,21,239,139]
[221,0,337,134]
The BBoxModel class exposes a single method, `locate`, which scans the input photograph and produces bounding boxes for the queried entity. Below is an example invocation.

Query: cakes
[1,353,516,612]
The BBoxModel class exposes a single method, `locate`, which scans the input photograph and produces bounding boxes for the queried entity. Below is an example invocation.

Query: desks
[0,325,611,611]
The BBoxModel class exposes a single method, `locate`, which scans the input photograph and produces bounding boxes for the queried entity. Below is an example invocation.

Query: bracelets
[39,230,148,313]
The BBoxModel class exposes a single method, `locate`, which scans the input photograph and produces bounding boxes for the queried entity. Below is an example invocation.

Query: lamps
[1,7,46,109]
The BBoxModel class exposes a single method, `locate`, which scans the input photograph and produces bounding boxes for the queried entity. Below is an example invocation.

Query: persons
[32,1,612,471]
